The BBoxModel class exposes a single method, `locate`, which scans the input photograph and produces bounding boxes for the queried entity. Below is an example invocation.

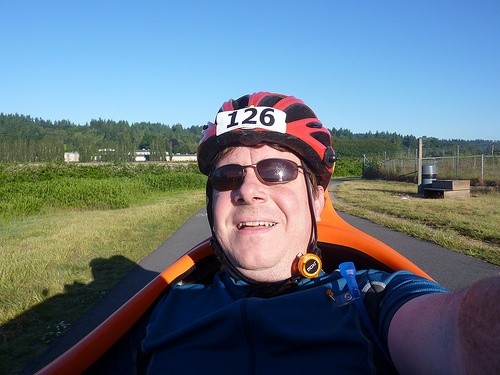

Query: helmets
[197,92,337,191]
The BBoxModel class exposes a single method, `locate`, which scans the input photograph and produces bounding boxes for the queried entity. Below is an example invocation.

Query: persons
[98,91,500,375]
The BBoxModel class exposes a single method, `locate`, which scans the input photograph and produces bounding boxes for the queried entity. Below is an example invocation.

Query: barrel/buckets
[422,164,437,184]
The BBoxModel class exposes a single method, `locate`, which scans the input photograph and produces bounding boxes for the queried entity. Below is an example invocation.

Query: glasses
[208,158,313,191]
